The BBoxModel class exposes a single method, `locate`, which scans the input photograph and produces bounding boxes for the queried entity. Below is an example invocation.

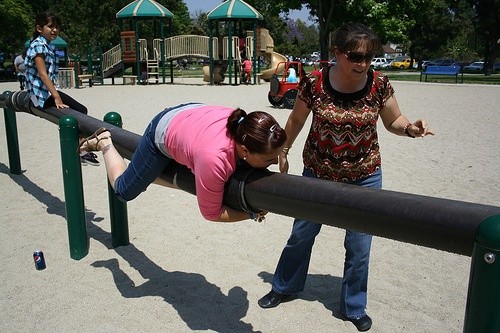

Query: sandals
[78,127,112,153]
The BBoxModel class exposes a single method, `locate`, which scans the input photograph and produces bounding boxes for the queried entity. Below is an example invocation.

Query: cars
[428,59,454,66]
[463,61,484,71]
[304,52,336,67]
[380,60,396,70]
[370,58,387,71]
[413,61,430,69]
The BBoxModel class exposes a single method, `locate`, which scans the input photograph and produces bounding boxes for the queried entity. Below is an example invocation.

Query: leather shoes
[352,314,372,331]
[258,290,290,308]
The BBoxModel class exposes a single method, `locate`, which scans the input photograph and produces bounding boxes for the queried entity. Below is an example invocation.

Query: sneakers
[80,153,100,166]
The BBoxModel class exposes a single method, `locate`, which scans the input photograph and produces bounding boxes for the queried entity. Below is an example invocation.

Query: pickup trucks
[392,58,415,70]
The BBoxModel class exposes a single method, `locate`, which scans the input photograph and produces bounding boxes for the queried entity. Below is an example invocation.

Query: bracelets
[404,122,415,139]
[250,211,254,219]
[282,148,289,154]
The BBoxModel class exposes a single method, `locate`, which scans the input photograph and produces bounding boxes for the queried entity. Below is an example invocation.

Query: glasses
[338,49,375,63]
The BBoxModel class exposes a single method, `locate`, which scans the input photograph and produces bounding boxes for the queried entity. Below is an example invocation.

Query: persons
[297,69,311,89]
[240,58,251,83]
[286,66,297,83]
[258,21,434,331]
[14,52,30,90]
[26,12,100,165]
[78,101,287,222]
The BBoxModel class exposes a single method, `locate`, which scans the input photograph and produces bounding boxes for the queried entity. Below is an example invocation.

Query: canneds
[33,251,46,270]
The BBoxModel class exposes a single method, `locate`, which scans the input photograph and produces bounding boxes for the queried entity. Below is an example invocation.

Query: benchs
[420,66,463,84]
